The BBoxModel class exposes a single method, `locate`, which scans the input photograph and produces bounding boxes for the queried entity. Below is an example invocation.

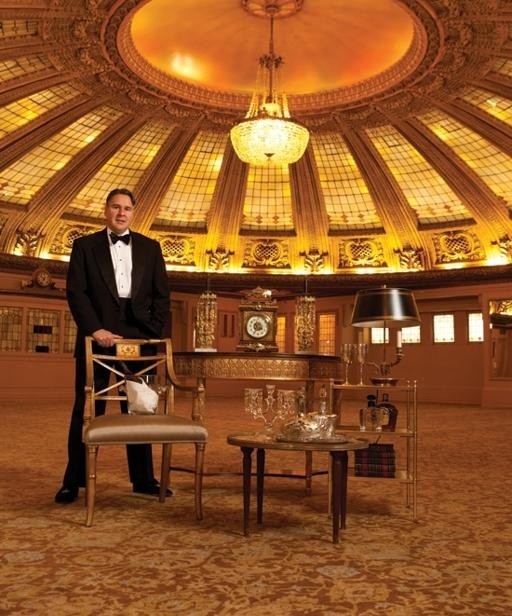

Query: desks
[225,433,369,546]
[157,350,342,502]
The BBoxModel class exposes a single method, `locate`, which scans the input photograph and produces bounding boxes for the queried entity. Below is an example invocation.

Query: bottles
[359,392,398,431]
[241,384,337,442]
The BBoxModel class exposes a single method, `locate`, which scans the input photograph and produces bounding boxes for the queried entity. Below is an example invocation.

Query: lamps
[349,287,422,386]
[228,0,310,169]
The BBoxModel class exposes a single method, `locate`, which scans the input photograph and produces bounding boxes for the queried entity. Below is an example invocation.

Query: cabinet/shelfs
[323,375,420,522]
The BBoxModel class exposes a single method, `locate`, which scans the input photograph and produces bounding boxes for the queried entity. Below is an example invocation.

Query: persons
[54,187,172,503]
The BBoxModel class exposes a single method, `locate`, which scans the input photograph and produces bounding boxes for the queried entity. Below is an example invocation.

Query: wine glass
[340,343,354,385]
[355,343,368,385]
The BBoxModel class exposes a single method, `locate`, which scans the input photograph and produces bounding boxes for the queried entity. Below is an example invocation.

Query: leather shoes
[54,485,79,504]
[132,483,172,497]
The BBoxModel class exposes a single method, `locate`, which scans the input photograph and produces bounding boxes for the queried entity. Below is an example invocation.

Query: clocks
[234,286,280,354]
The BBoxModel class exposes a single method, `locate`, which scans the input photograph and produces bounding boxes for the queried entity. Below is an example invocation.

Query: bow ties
[111,232,130,245]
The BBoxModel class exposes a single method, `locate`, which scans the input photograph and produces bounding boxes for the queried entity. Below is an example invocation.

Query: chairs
[78,336,207,528]
